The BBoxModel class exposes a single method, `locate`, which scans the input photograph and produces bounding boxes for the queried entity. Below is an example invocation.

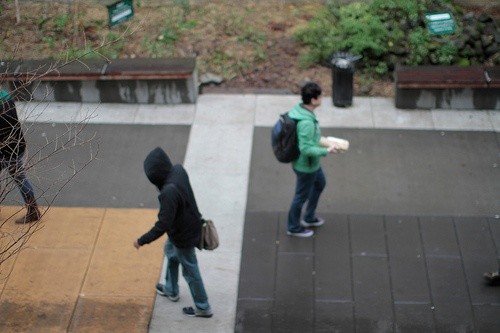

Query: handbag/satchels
[194,218,220,251]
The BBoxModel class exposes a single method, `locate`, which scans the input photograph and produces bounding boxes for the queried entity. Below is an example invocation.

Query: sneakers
[300,218,325,226]
[182,306,214,319]
[156,282,181,302]
[287,227,314,238]
[14,212,41,224]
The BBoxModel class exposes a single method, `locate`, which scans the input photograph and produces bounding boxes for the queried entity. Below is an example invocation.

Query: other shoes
[483,271,500,282]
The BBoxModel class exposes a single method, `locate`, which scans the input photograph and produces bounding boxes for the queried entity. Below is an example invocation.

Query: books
[320,135,349,154]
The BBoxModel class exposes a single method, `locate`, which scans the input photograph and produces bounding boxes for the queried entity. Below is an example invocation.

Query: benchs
[395,66,499,108]
[1,56,198,104]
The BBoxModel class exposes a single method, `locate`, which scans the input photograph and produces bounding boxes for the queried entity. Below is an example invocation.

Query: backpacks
[270,111,302,164]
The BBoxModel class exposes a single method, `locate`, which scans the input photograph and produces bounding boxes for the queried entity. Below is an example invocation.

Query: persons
[285,81,341,238]
[0,86,42,223]
[482,269,500,283]
[132,148,215,318]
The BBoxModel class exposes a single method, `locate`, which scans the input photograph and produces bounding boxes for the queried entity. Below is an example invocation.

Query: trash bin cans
[332,53,353,107]
